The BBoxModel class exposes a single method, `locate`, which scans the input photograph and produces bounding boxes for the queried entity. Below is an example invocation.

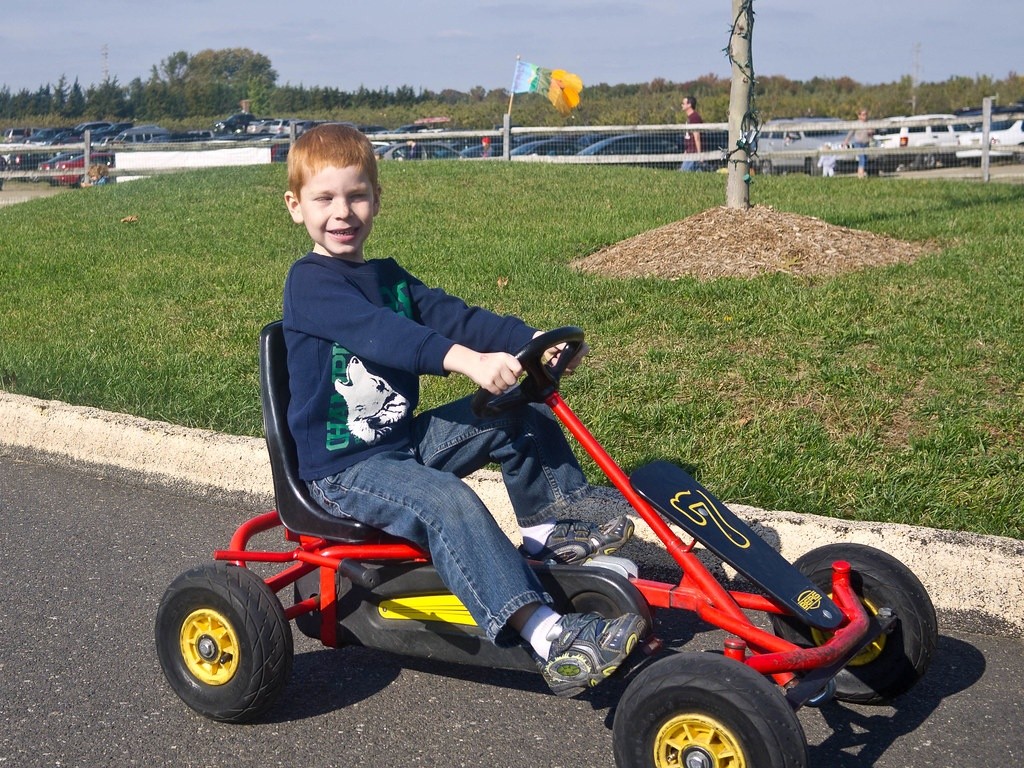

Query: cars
[0,111,1024,183]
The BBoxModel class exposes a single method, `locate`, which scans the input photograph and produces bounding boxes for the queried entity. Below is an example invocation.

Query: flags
[511,60,584,112]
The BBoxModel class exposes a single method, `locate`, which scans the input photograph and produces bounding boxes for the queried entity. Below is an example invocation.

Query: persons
[406,141,423,160]
[479,136,495,157]
[675,95,708,170]
[816,139,839,176]
[79,163,111,187]
[272,122,645,702]
[842,107,876,177]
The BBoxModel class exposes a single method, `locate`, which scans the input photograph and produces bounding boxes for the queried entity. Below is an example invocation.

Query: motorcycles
[153,317,939,767]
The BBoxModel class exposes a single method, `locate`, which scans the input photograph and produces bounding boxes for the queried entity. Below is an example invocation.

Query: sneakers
[516,515,635,567]
[531,611,646,698]
